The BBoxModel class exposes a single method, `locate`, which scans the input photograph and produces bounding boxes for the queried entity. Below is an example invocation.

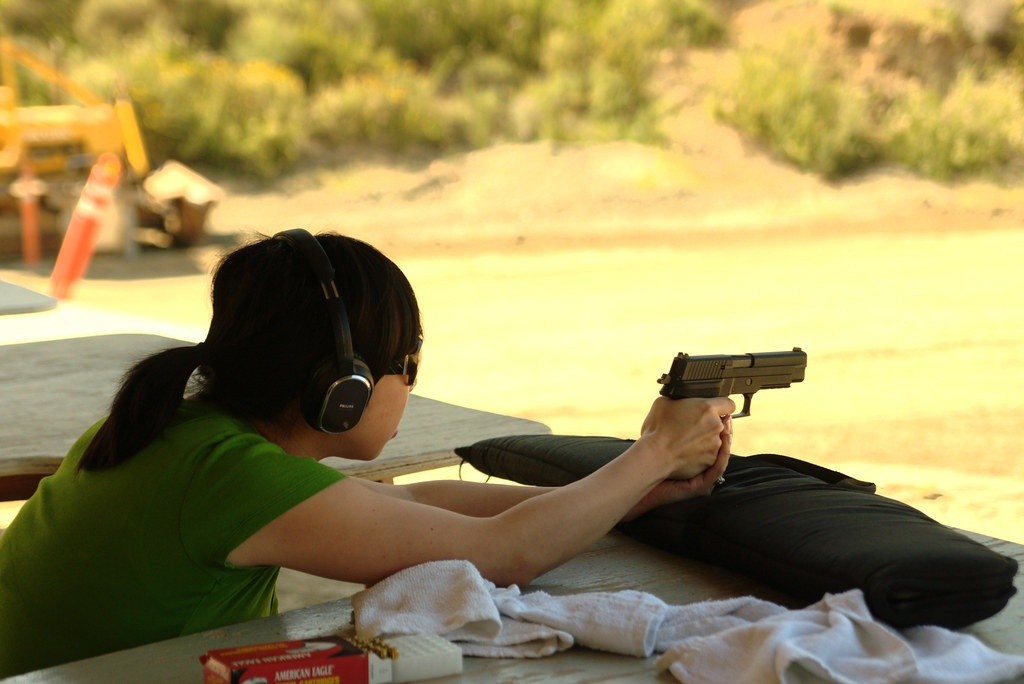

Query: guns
[655,345,809,420]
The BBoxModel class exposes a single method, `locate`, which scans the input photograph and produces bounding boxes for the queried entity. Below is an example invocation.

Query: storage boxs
[200,635,369,684]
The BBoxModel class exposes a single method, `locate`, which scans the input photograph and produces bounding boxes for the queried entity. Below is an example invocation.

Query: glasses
[356,324,424,386]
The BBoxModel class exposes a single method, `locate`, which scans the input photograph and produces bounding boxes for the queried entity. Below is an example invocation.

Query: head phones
[272,229,374,434]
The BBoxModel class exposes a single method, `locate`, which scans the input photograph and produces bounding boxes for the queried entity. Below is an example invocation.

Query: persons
[0,230,735,680]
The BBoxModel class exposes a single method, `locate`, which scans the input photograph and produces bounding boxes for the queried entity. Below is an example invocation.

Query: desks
[0,526,1024,684]
[1,333,551,501]
[0,280,59,314]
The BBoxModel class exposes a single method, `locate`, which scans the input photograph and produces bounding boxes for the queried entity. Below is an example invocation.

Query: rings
[717,478,725,483]
